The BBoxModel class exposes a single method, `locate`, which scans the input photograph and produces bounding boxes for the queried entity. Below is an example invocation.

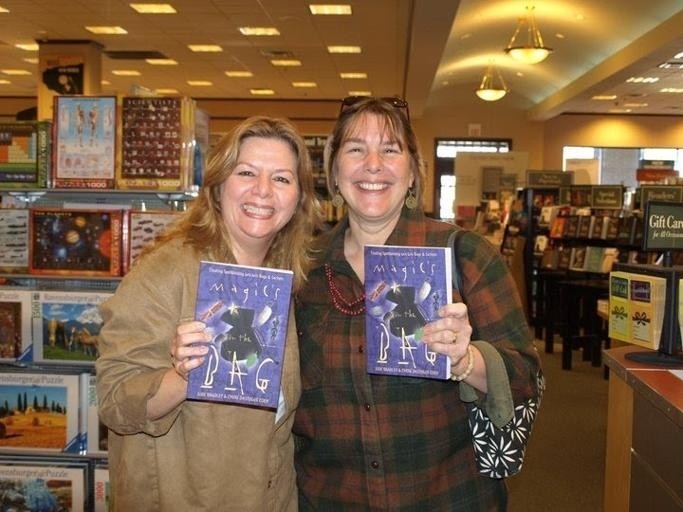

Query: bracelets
[443,346,476,384]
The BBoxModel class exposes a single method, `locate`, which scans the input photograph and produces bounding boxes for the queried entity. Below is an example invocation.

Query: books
[607,268,668,353]
[361,242,452,386]
[181,258,296,414]
[474,186,654,273]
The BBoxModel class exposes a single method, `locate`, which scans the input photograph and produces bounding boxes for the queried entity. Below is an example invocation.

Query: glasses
[338,94,411,128]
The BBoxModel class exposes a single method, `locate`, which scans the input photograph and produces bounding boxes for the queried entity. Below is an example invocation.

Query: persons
[290,92,546,511]
[93,116,329,511]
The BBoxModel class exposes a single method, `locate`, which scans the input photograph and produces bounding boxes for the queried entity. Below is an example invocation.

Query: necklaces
[320,260,366,318]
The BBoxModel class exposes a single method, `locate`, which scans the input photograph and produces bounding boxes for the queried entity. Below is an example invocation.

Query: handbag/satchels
[461,363,547,482]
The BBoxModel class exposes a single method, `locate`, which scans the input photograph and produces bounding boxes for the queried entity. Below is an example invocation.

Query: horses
[48,319,99,357]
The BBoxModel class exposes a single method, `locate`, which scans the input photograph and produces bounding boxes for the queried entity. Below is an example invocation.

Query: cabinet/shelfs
[0,182,200,511]
[515,183,561,328]
[536,212,649,276]
[593,344,681,511]
[560,184,592,212]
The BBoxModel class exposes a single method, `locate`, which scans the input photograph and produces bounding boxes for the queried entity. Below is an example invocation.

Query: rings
[451,332,457,343]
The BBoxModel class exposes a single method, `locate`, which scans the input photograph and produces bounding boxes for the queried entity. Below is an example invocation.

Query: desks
[543,273,613,382]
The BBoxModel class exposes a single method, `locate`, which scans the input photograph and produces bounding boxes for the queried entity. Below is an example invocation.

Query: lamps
[474,64,509,103]
[499,5,555,67]
